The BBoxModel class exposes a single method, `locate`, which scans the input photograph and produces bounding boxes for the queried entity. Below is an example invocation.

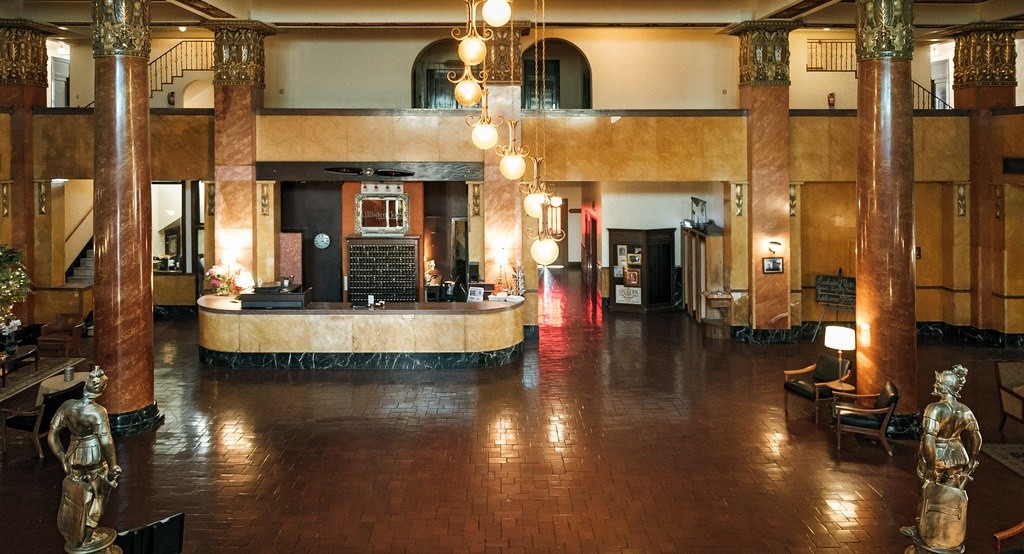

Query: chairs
[0,313,86,459]
[116,512,185,554]
[992,358,1024,432]
[783,353,899,456]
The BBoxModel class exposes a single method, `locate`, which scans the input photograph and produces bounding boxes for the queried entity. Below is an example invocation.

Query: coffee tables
[0,345,38,388]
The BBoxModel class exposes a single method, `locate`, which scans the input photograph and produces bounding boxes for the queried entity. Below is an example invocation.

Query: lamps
[824,325,856,390]
[768,241,782,254]
[446,0,566,266]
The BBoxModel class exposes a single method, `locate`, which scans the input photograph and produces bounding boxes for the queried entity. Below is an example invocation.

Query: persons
[899,364,983,537]
[47,366,119,548]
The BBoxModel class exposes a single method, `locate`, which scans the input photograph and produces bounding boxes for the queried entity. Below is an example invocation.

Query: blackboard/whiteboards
[816,275,856,307]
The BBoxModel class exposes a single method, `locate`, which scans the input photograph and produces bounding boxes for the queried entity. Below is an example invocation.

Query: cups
[283,279,289,287]
[368,294,374,305]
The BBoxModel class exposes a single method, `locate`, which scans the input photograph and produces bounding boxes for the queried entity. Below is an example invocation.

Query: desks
[240,284,313,309]
[36,372,92,406]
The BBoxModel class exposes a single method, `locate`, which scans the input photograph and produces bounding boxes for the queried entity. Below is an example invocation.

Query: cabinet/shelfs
[681,223,724,322]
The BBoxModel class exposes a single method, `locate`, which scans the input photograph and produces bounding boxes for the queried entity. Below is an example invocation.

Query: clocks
[315,234,330,249]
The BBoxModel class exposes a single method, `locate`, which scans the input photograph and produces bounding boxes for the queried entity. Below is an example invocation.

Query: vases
[5,332,18,355]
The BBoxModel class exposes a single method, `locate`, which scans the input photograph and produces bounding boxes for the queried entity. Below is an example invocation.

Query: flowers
[0,243,33,336]
[205,263,241,295]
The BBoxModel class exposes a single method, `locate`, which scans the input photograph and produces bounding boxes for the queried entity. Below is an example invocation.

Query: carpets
[0,357,87,402]
[980,443,1024,478]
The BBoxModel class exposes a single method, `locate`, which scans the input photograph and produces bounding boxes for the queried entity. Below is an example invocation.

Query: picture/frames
[916,247,921,259]
[613,244,641,288]
[762,257,785,275]
